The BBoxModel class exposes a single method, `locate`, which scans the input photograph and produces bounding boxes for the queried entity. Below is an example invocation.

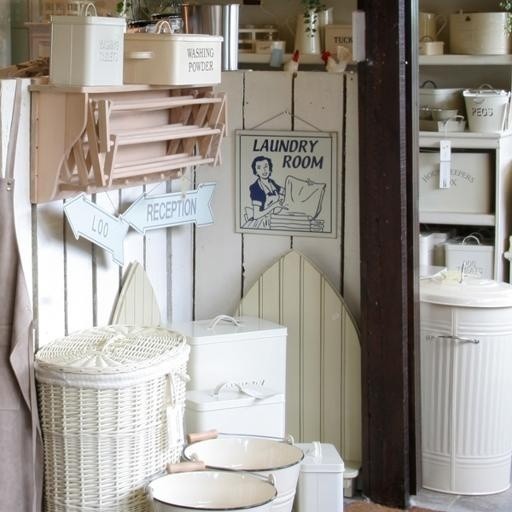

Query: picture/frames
[235,130,338,238]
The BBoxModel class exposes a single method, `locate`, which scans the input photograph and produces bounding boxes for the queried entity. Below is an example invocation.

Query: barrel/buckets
[186,435,305,511]
[464,82,508,133]
[148,471,281,510]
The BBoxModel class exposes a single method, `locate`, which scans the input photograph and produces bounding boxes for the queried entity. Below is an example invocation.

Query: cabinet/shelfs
[0,69,367,511]
[409,0,510,280]
[29,88,228,208]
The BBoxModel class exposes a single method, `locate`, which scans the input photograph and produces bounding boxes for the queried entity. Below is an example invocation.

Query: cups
[286,7,333,54]
[419,11,448,42]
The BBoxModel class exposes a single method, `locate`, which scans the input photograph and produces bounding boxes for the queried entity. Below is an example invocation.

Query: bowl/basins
[418,108,459,124]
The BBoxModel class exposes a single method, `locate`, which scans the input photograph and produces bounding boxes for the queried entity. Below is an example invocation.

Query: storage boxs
[418,88,463,110]
[45,14,223,87]
[444,232,494,279]
[183,381,287,444]
[185,316,290,392]
[295,440,345,511]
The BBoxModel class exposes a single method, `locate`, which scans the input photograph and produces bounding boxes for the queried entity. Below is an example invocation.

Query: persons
[249,155,289,219]
